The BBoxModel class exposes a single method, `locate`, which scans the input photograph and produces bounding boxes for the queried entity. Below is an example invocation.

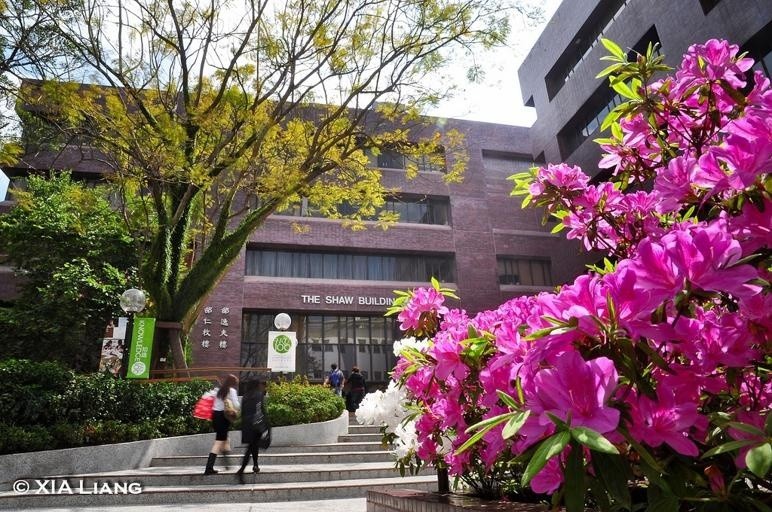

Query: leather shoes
[236,470,245,485]
[252,466,260,471]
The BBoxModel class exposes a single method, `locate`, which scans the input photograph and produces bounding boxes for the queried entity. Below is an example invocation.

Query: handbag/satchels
[224,399,237,420]
[193,395,213,421]
[254,414,271,449]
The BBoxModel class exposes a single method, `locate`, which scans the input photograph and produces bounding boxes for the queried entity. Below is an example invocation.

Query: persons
[232,377,272,484]
[345,365,367,411]
[195,373,241,474]
[323,362,345,398]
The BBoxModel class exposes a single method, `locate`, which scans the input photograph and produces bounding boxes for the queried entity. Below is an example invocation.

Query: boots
[205,452,217,475]
